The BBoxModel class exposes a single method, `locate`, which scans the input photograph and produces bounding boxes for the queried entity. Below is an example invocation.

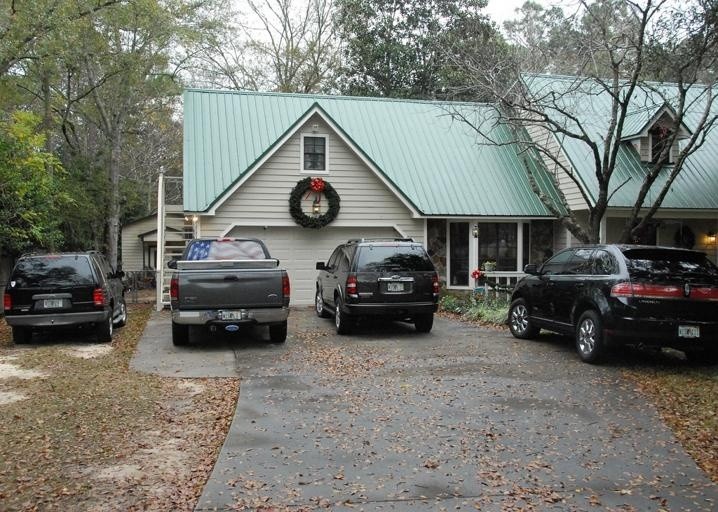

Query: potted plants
[480,256,496,271]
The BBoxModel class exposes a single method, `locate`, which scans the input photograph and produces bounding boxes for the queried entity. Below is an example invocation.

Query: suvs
[3,248,128,345]
[312,235,440,335]
[505,243,716,369]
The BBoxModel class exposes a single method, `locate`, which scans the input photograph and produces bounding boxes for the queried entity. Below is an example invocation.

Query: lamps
[705,230,717,243]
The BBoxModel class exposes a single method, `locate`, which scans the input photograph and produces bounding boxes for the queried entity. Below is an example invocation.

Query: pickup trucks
[167,234,291,346]
